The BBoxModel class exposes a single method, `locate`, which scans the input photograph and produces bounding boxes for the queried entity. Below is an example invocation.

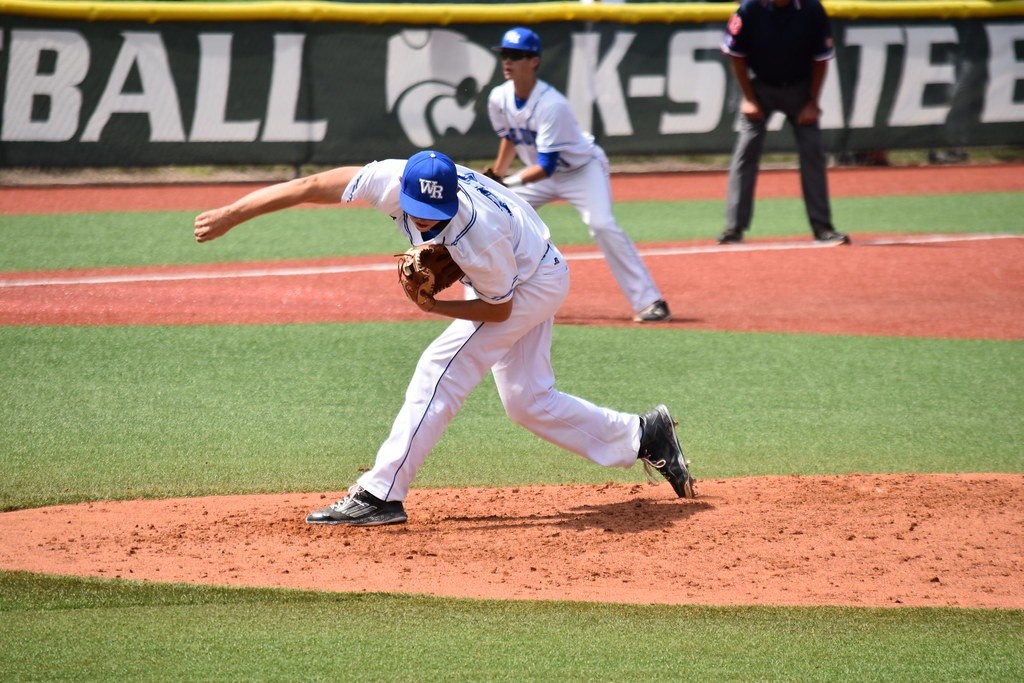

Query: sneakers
[634,300,671,322]
[638,404,698,498]
[306,485,407,526]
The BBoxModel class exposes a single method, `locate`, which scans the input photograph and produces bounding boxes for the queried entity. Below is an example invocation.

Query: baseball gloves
[399,240,464,308]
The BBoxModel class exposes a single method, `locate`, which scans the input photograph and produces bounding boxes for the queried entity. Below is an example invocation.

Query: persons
[483,28,673,323]
[715,0,850,246]
[193,150,695,527]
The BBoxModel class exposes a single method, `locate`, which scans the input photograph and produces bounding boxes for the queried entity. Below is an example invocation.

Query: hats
[491,27,542,54]
[399,150,459,220]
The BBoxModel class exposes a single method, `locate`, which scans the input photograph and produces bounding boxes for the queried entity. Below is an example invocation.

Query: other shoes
[816,228,849,246]
[833,149,889,166]
[927,149,968,165]
[717,228,741,244]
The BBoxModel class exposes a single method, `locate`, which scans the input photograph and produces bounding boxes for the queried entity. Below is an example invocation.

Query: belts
[541,244,550,260]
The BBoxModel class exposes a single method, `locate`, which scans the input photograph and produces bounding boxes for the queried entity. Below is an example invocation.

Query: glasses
[500,50,537,61]
